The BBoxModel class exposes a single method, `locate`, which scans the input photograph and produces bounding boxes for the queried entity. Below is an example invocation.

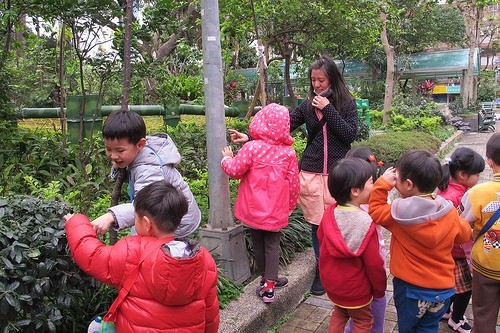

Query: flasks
[87,316,115,333]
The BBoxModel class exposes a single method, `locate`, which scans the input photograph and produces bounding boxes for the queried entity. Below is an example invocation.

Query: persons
[221,103,300,302]
[228,59,358,296]
[63,180,219,333]
[89,108,202,242]
[368,150,471,333]
[316,147,388,333]
[296,94,302,99]
[437,147,485,333]
[457,132,500,333]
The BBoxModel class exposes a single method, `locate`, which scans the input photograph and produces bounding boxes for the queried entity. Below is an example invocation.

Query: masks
[314,85,333,99]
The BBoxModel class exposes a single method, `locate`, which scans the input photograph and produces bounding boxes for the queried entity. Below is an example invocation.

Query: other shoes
[442,306,465,319]
[448,316,472,333]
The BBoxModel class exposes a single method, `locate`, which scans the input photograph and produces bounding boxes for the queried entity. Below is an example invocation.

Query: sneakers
[311,272,326,294]
[256,286,276,302]
[259,277,288,289]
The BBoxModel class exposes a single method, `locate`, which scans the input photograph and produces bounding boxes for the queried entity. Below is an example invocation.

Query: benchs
[478,109,496,133]
